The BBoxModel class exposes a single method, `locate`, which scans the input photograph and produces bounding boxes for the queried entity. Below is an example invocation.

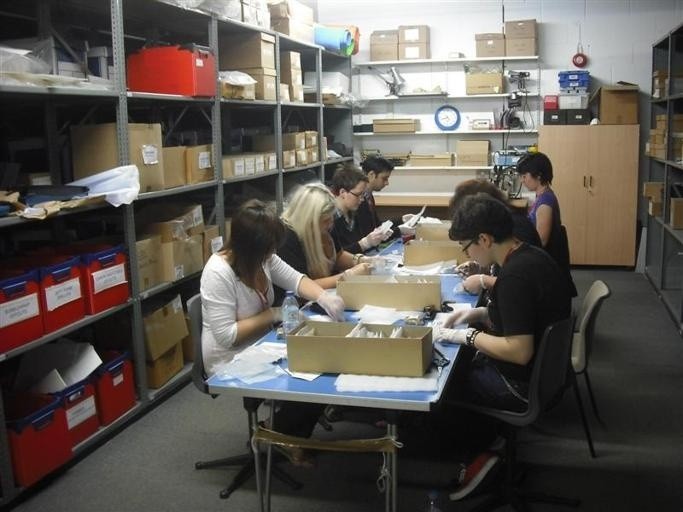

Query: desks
[205,238,494,510]
[366,193,528,227]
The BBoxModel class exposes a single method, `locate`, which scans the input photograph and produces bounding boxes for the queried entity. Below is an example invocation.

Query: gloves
[342,263,375,276]
[441,306,488,328]
[316,291,345,323]
[433,326,469,347]
[268,305,309,323]
[463,273,487,297]
[454,261,490,280]
[397,213,420,236]
[362,227,394,249]
[361,254,388,269]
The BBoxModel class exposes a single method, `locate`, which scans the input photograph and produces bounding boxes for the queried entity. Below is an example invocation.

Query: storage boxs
[337,274,443,312]
[401,240,465,267]
[643,69,682,232]
[414,222,449,240]
[361,17,539,169]
[541,65,591,123]
[1,2,330,492]
[286,320,432,378]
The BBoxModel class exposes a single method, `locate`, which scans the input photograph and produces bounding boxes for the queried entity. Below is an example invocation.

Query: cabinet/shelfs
[0,0,353,511]
[350,55,540,198]
[589,85,639,124]
[639,18,682,338]
[541,125,637,266]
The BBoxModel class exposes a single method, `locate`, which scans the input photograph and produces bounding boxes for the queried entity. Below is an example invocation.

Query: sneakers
[486,433,507,452]
[447,449,500,501]
[258,419,315,468]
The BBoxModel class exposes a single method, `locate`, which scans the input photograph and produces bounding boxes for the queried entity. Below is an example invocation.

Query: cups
[492,105,510,129]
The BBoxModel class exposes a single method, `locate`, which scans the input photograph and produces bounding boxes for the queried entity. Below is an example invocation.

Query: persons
[446,179,542,307]
[510,151,560,255]
[200,199,347,476]
[417,192,571,500]
[271,180,381,423]
[328,163,394,253]
[356,154,416,240]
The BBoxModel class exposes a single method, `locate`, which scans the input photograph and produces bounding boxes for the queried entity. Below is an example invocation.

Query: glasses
[462,238,477,258]
[346,189,368,199]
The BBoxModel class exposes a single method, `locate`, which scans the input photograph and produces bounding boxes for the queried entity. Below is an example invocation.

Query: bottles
[281,290,300,335]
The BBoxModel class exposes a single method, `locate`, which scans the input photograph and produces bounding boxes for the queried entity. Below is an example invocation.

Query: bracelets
[465,327,480,351]
[351,253,363,266]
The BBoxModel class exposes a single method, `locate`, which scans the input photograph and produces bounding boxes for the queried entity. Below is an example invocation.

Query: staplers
[423,305,435,320]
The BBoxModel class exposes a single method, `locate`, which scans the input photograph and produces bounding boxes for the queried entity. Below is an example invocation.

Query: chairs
[184,292,307,499]
[504,276,612,460]
[440,312,595,510]
